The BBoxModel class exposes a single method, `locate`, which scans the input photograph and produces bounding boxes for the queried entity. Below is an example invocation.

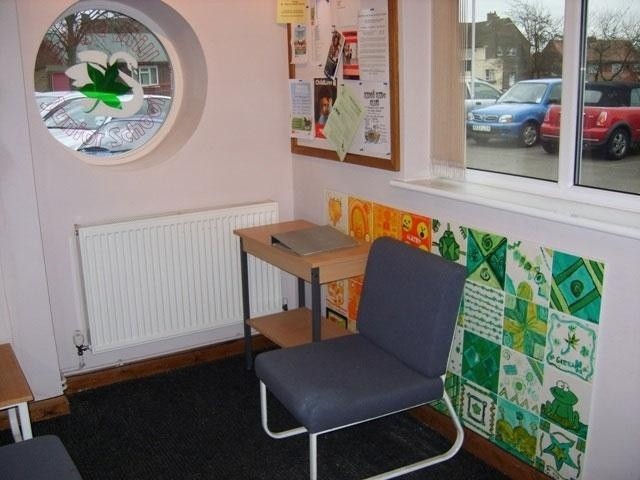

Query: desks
[0,344,36,444]
[234,218,373,380]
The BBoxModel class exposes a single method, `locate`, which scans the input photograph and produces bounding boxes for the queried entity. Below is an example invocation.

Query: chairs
[253,235,471,480]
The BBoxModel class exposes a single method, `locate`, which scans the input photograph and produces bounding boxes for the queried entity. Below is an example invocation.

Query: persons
[315,87,334,126]
[329,33,353,65]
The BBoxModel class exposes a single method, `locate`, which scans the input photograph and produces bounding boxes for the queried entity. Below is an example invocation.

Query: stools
[0,433,81,480]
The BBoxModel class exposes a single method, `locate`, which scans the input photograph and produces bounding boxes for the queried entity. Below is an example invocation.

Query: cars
[539,81,637,159]
[465,77,504,111]
[469,79,555,147]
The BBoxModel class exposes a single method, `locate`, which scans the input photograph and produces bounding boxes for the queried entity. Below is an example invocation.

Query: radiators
[74,198,286,367]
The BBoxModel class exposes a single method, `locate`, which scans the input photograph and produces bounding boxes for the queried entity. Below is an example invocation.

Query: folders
[270,224,359,257]
